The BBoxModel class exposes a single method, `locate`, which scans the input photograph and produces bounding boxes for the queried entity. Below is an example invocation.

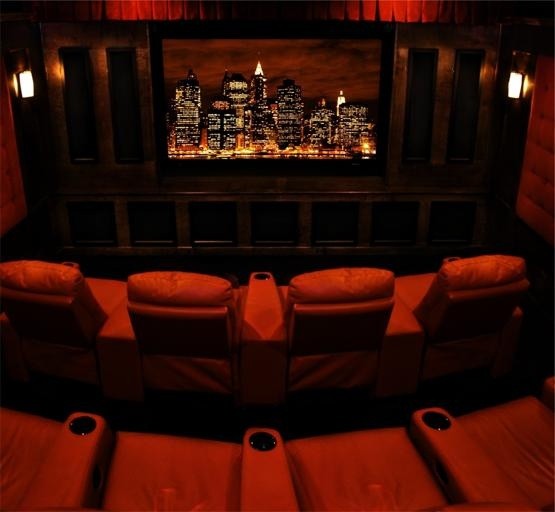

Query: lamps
[7,52,39,113]
[503,49,532,110]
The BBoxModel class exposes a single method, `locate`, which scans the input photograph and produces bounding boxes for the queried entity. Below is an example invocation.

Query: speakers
[106,46,144,165]
[445,47,484,163]
[400,45,438,165]
[57,45,101,166]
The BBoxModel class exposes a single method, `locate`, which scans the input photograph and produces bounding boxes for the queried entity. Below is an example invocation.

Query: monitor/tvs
[146,16,397,178]
